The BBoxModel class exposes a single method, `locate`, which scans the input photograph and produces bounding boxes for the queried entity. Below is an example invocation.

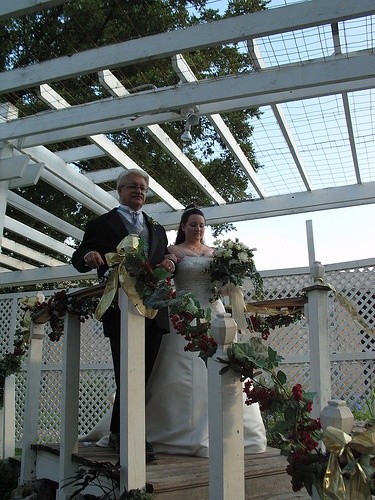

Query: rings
[168,267,171,269]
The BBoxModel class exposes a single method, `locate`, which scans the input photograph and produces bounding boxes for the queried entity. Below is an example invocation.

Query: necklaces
[184,242,202,256]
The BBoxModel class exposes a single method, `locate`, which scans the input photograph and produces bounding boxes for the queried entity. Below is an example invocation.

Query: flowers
[238,292,307,341]
[148,216,159,226]
[202,238,264,303]
[0,288,118,410]
[125,236,217,369]
[216,341,375,500]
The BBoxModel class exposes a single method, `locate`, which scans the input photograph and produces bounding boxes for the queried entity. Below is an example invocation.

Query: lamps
[181,122,193,141]
[180,107,200,126]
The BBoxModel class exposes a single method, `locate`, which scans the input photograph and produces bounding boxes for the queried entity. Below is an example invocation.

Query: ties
[119,207,144,233]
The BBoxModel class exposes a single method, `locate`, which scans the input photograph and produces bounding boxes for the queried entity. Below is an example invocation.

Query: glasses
[119,184,151,192]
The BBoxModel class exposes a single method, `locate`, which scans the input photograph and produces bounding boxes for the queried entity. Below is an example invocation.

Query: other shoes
[145,442,160,463]
[109,433,120,451]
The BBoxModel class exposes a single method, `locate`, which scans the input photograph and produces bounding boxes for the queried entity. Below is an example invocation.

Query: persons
[77,208,269,459]
[71,169,176,468]
